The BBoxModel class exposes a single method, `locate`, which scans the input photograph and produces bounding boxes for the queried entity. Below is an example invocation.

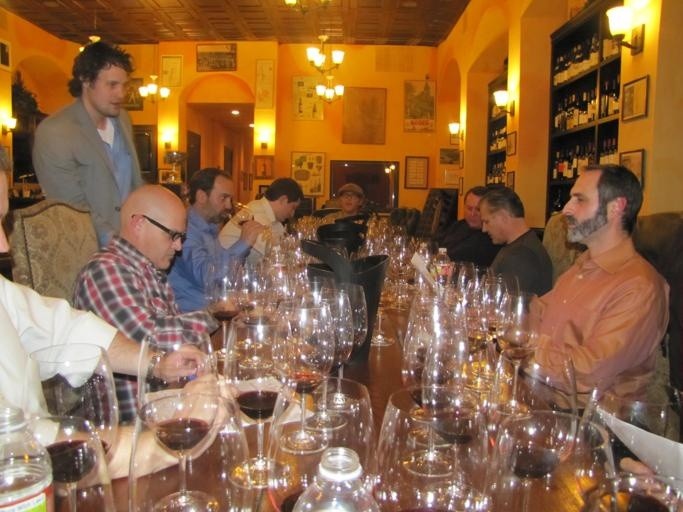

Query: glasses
[131,214,186,243]
[343,193,358,199]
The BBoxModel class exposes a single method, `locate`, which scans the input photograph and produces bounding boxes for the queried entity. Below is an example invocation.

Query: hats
[337,183,363,196]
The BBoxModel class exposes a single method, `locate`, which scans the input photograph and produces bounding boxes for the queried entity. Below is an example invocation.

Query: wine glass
[28,212,683,512]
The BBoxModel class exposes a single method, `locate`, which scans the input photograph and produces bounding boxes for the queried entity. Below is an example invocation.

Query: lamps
[1,108,18,135]
[283,0,332,15]
[606,6,646,55]
[493,90,517,115]
[306,28,347,72]
[79,0,101,52]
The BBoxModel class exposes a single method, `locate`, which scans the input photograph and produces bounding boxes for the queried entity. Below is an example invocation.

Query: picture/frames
[619,149,646,190]
[506,131,517,156]
[0,38,10,70]
[622,75,649,121]
[507,171,515,192]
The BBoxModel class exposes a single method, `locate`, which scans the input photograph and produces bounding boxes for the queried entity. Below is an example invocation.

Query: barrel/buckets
[314,214,372,253]
[302,236,391,363]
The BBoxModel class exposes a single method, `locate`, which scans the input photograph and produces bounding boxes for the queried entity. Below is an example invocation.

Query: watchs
[147,347,167,383]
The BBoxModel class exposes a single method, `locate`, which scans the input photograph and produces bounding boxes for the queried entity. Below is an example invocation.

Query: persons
[470,162,671,414]
[436,184,498,265]
[32,42,146,250]
[1,149,207,506]
[218,178,304,265]
[321,182,368,220]
[167,166,265,315]
[475,187,553,301]
[73,182,248,430]
[612,379,683,512]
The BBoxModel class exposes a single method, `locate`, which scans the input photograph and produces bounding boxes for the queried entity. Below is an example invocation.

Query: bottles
[554,73,620,133]
[1,407,54,512]
[552,135,618,182]
[489,123,506,151]
[486,157,506,184]
[553,35,598,77]
